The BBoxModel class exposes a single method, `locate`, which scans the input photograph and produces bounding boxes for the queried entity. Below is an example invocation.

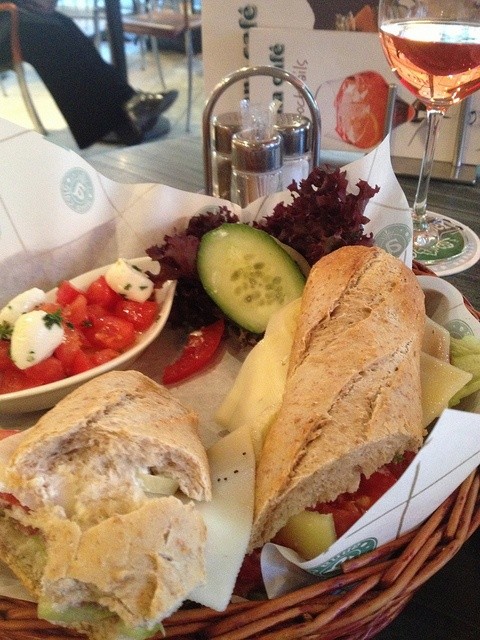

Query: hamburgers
[1,370,257,640]
[215,245,479,555]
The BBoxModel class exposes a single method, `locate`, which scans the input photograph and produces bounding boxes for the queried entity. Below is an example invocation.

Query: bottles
[230,132,285,209]
[213,116,253,201]
[270,113,311,191]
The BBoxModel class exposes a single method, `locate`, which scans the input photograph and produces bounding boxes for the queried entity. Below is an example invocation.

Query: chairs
[0,0,50,137]
[91,0,203,133]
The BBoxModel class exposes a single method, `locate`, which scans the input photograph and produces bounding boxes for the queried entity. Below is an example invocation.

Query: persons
[0,0,180,150]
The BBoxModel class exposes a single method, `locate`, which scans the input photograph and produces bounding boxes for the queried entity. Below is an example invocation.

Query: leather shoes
[122,89,177,145]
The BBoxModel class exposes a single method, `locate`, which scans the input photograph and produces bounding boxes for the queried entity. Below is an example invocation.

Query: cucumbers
[198,222,303,336]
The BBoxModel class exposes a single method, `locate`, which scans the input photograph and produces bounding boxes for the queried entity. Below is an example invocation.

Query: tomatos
[164,316,226,385]
[0,275,160,393]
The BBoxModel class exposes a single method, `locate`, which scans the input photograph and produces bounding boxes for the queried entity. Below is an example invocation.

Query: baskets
[0,261,480,636]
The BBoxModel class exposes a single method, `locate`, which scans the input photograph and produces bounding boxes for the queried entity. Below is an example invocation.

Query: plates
[0,254,177,414]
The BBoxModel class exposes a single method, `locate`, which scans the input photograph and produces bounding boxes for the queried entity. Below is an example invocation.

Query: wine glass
[376,1,480,276]
[311,71,444,149]
